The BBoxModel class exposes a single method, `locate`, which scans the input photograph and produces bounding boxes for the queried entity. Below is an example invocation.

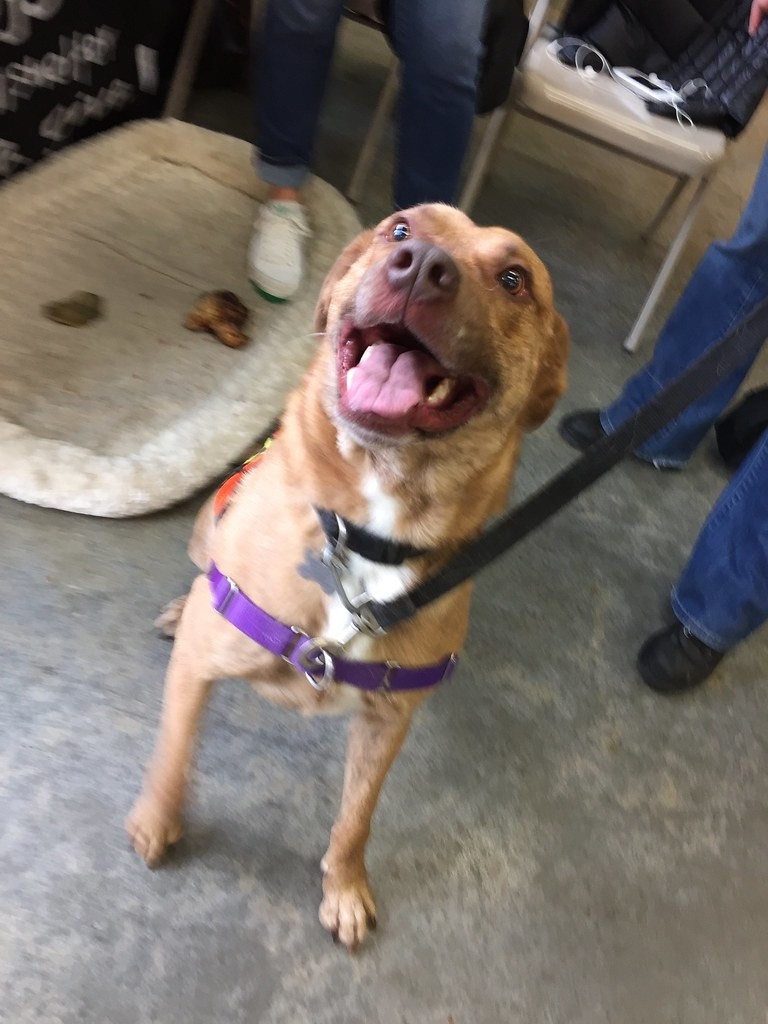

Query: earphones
[584,65,600,80]
[649,73,667,88]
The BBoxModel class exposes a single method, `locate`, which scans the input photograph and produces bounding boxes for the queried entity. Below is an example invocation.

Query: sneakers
[560,411,682,471]
[248,197,316,303]
[638,623,725,691]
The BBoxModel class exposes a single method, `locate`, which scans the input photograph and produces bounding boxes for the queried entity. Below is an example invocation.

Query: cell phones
[613,66,683,105]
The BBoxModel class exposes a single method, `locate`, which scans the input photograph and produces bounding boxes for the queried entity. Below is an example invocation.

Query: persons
[248,0,486,303]
[561,0,767,691]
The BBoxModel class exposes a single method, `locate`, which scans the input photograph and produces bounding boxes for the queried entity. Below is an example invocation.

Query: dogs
[127,205,568,952]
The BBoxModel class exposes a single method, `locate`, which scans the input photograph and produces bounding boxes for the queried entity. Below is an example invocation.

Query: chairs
[160,0,407,204]
[456,0,754,355]
